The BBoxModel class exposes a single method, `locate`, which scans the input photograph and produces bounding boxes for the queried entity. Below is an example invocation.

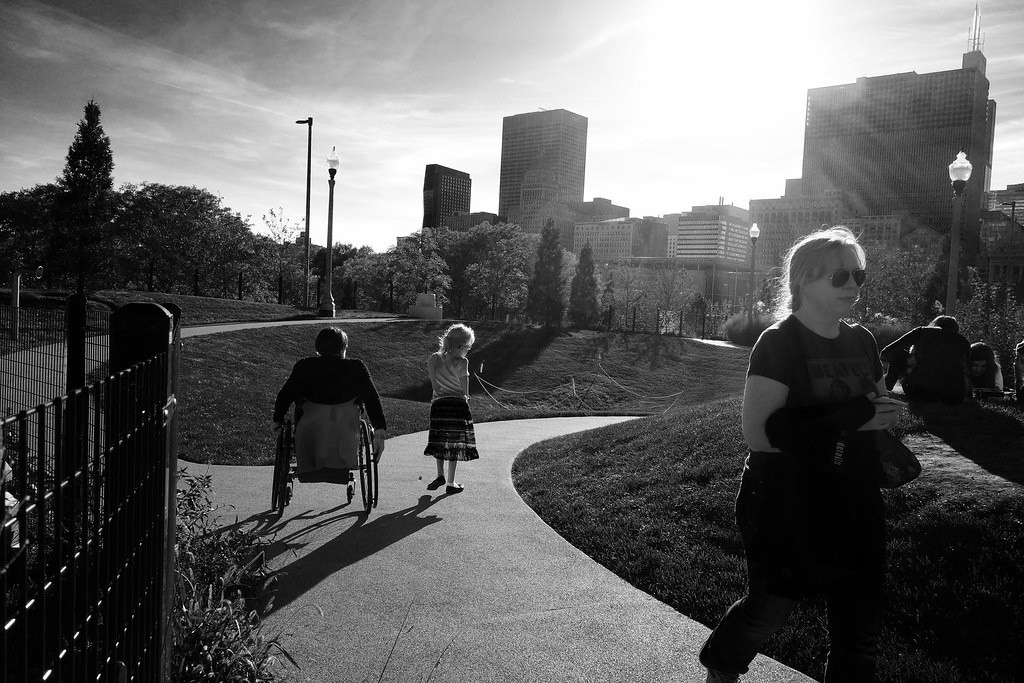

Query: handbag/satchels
[872,428,921,489]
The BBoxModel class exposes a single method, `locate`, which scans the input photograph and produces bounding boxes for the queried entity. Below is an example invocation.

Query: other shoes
[446,484,463,494]
[427,476,446,490]
[706,668,741,683]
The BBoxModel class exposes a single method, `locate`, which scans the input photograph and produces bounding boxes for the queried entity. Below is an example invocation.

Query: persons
[879,316,1003,399]
[424,323,479,493]
[271,328,386,463]
[698,225,907,683]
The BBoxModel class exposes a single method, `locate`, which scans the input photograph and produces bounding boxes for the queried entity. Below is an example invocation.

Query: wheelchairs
[270,396,378,516]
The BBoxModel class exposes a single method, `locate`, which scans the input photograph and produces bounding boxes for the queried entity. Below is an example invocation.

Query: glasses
[820,270,866,288]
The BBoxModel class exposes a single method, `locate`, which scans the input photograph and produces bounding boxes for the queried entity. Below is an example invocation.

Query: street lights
[948,153,972,316]
[748,224,760,329]
[326,150,338,296]
[297,119,312,302]
